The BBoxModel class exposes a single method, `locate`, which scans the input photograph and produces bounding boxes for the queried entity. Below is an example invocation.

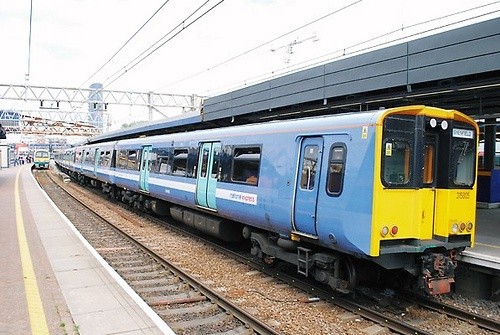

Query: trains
[54,104,480,296]
[33,149,50,170]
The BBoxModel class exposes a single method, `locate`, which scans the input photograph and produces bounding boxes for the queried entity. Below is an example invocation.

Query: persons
[242,162,259,183]
[13,154,32,166]
